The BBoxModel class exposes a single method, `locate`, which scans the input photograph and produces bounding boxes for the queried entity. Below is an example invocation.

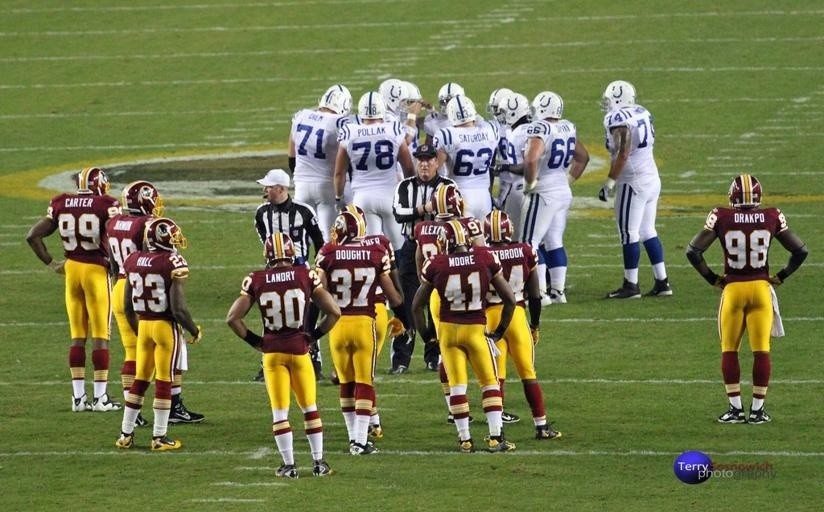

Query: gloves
[48,259,65,275]
[531,327,539,345]
[599,184,611,201]
[522,177,537,192]
[334,196,346,213]
[387,316,411,337]
[425,338,442,355]
[186,326,202,344]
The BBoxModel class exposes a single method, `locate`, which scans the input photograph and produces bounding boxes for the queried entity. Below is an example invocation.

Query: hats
[412,144,437,158]
[256,168,290,187]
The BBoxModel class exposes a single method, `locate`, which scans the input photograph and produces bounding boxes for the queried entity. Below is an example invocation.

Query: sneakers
[428,360,438,371]
[607,277,642,299]
[718,403,746,424]
[448,410,562,452]
[350,424,383,456]
[388,364,409,374]
[312,458,332,477]
[524,289,567,307]
[643,277,673,296]
[71,393,205,452]
[747,402,772,424]
[275,464,298,480]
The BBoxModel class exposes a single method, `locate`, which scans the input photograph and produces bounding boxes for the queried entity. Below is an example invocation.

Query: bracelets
[311,328,322,338]
[494,324,504,333]
[244,331,259,347]
[704,270,717,284]
[776,268,788,281]
[422,331,430,339]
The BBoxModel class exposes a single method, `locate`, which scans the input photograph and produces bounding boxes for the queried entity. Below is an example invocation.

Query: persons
[107,179,203,426]
[414,185,519,423]
[686,173,808,423]
[115,219,200,452]
[26,168,123,412]
[597,81,673,301]
[287,79,591,306]
[411,221,516,452]
[311,204,403,437]
[483,209,563,440]
[226,232,340,479]
[386,145,461,375]
[316,213,413,456]
[250,169,324,383]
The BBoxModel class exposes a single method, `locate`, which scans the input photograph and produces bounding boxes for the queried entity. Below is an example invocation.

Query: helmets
[728,175,762,207]
[121,181,162,217]
[264,232,295,264]
[318,79,423,120]
[437,82,476,126]
[489,88,563,129]
[600,81,635,111]
[144,218,188,253]
[332,206,367,246]
[431,185,514,255]
[76,167,110,196]
